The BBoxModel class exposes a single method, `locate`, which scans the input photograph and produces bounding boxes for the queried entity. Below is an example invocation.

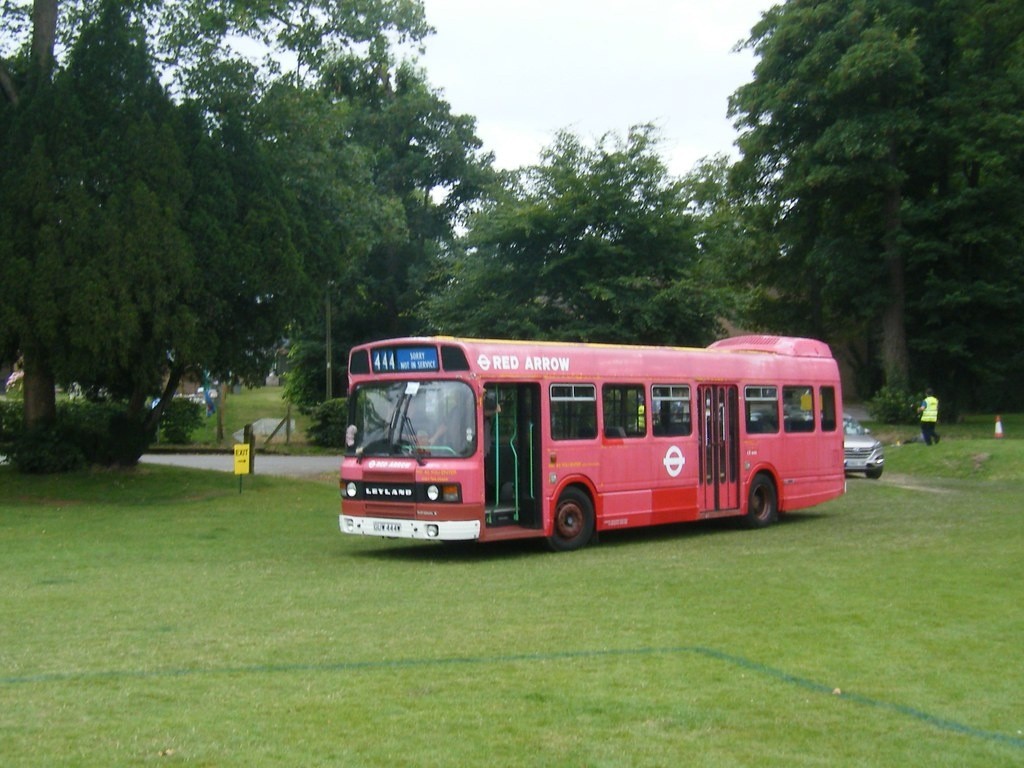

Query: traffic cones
[995,416,1005,439]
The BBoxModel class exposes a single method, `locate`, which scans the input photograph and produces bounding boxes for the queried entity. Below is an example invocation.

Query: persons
[918,388,940,445]
[386,400,436,442]
[429,386,501,507]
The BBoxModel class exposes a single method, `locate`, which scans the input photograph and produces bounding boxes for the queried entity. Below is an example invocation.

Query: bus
[338,335,848,549]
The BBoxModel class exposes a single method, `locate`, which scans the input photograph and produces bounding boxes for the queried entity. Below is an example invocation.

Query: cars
[789,411,885,479]
